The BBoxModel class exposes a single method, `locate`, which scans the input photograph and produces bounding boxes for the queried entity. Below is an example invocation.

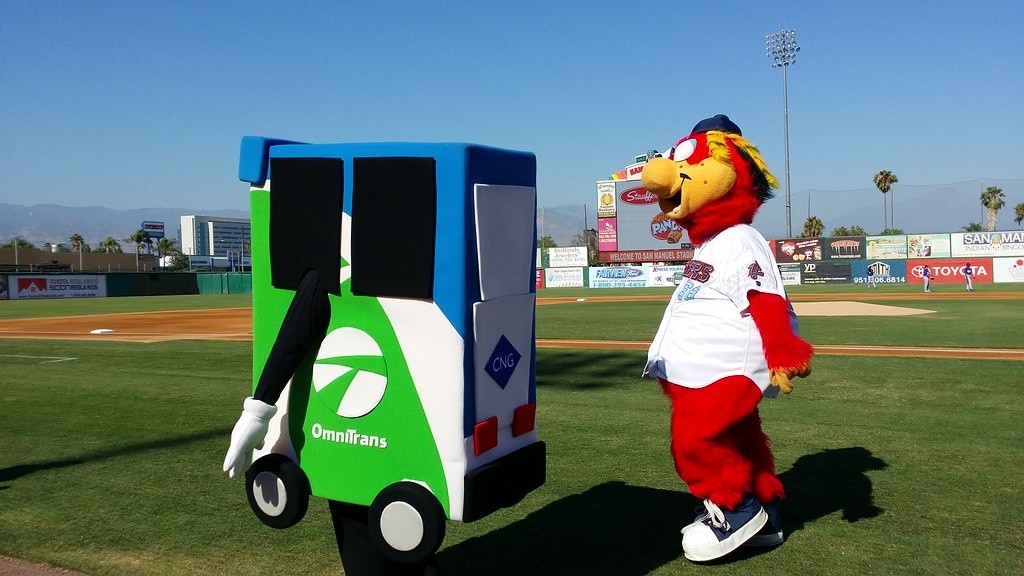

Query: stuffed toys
[642,113,814,560]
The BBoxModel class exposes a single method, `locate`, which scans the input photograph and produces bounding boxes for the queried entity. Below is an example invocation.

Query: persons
[866,266,876,289]
[964,263,974,292]
[923,265,931,293]
[777,265,782,279]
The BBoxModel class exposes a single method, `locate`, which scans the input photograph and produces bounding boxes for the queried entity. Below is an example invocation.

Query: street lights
[765,26,801,239]
